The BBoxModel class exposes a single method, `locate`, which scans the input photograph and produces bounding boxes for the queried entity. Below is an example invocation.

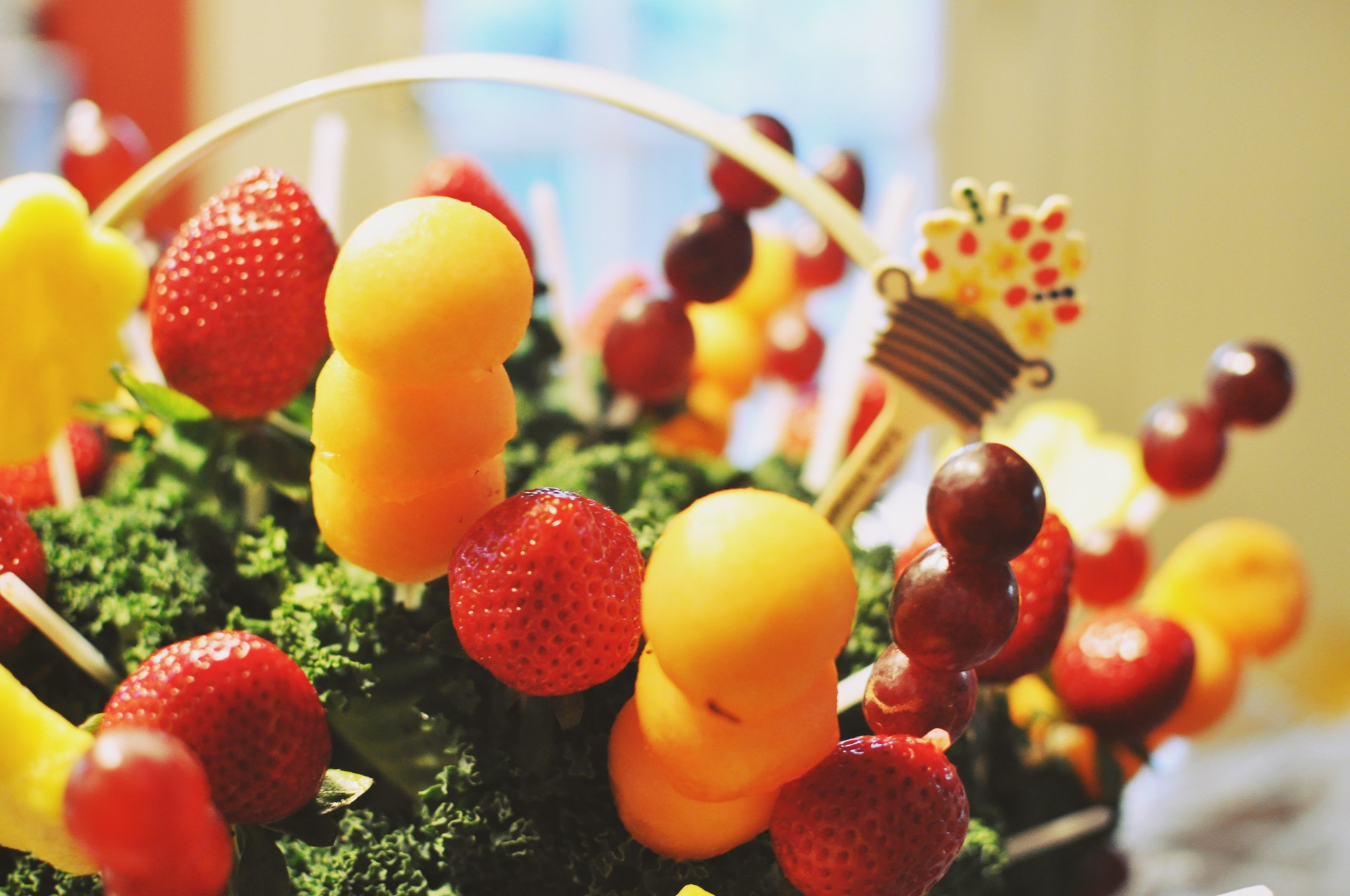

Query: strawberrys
[419,158,535,281]
[453,488,645,691]
[96,624,328,832]
[888,511,1073,676]
[1046,606,1191,740]
[0,419,102,642]
[147,167,337,417]
[765,727,970,896]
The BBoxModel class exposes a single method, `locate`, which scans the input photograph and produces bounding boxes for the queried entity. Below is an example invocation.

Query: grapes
[862,443,1053,753]
[604,111,794,401]
[56,96,196,241]
[1140,334,1294,495]
[64,722,234,894]
[754,147,870,383]
